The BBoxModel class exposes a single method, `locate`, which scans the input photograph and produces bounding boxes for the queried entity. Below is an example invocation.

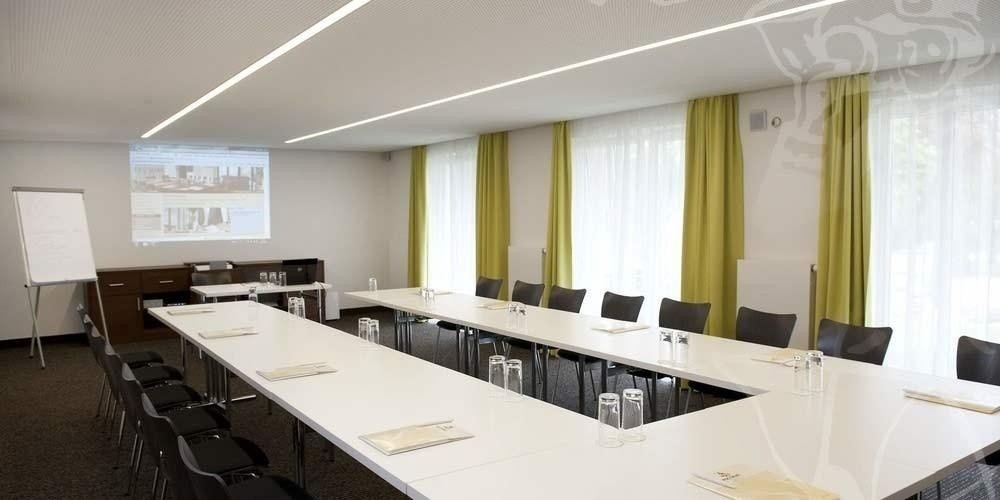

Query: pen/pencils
[417,417,455,427]
[692,471,736,489]
[901,386,937,398]
[298,360,328,366]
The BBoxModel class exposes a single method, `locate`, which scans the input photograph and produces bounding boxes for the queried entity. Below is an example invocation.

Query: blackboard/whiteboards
[11,186,98,287]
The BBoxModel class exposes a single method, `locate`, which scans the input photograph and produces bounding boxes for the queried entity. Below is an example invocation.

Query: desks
[146,285,1000,500]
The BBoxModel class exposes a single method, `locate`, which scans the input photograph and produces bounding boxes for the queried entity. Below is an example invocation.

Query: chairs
[503,284,587,395]
[69,304,207,499]
[469,280,547,379]
[815,318,894,366]
[684,306,798,412]
[133,393,309,500]
[558,290,646,414]
[955,332,1000,385]
[627,298,711,413]
[431,275,507,374]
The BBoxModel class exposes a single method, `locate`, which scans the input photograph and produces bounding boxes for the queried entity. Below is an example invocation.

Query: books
[257,362,337,382]
[413,287,451,295]
[168,305,216,315]
[904,377,1000,413]
[360,420,473,456]
[689,459,840,500]
[479,299,511,311]
[593,322,649,334]
[199,326,258,340]
[754,347,800,367]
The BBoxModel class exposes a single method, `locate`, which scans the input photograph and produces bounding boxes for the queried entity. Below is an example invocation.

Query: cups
[806,349,823,393]
[657,329,673,366]
[489,355,506,400]
[505,359,524,402]
[672,332,690,369]
[620,389,646,442]
[505,302,528,333]
[367,320,380,351]
[595,393,625,449]
[416,287,434,304]
[792,354,810,397]
[248,270,306,323]
[358,317,370,347]
[369,278,377,297]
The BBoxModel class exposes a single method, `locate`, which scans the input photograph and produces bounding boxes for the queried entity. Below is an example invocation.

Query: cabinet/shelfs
[81,258,332,343]
